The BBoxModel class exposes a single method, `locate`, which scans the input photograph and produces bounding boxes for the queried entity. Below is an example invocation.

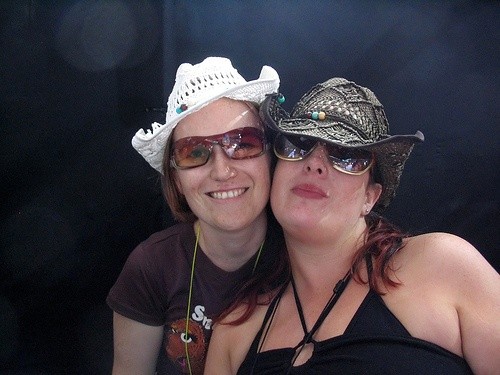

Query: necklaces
[185,225,267,375]
[251,256,359,375]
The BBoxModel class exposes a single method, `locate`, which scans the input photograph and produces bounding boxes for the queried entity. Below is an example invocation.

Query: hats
[130,57,280,174]
[258,76,423,210]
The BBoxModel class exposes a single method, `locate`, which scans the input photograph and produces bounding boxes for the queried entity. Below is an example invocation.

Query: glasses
[169,126,269,169]
[273,128,376,175]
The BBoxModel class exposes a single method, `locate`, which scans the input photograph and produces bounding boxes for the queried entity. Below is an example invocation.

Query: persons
[106,56,290,375]
[204,78,500,375]
